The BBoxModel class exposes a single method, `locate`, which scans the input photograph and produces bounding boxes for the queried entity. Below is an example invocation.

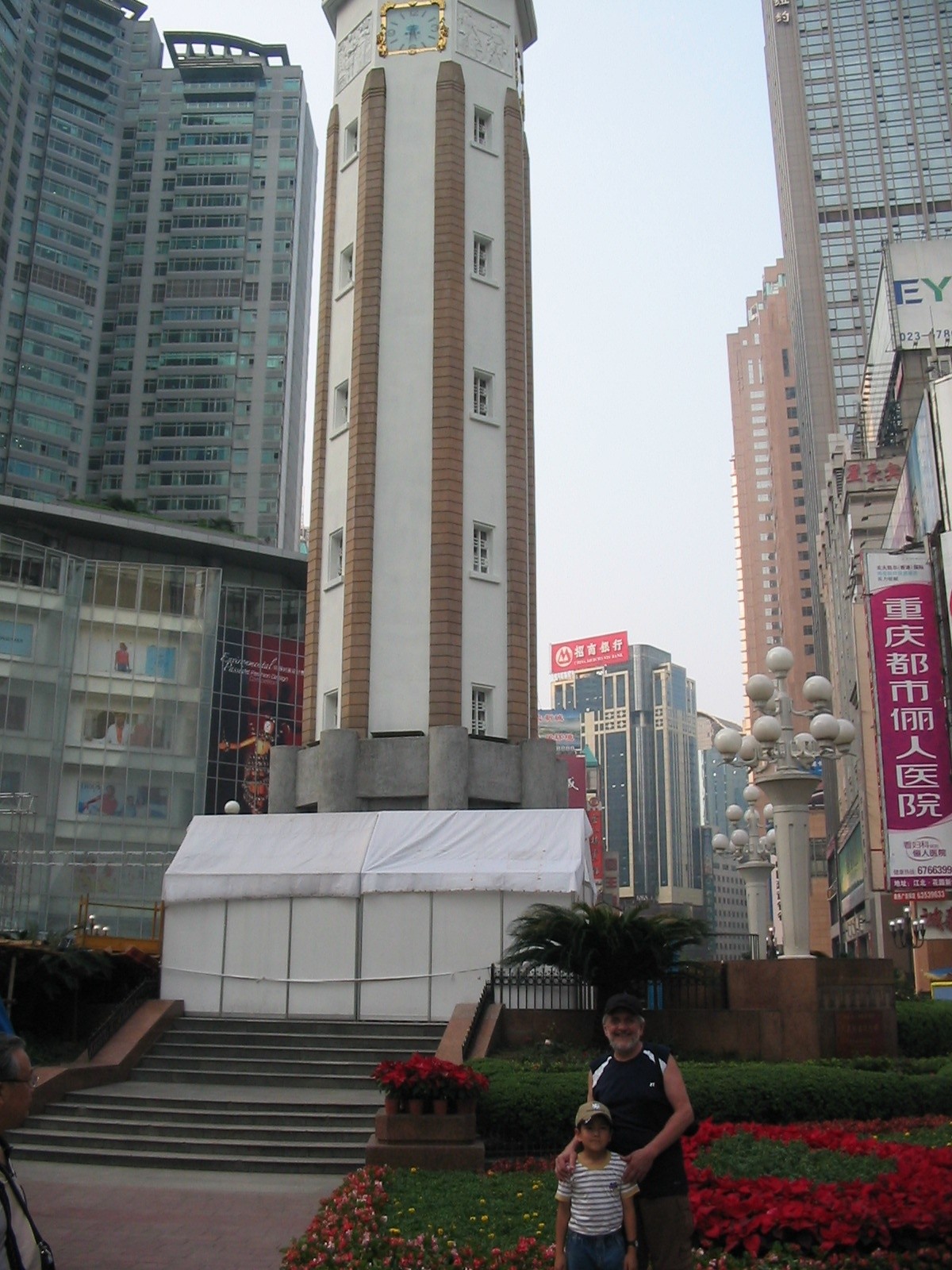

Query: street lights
[711,645,858,952]
[709,784,776,960]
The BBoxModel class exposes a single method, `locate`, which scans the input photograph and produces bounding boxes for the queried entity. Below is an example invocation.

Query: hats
[606,993,642,1015]
[576,1102,613,1129]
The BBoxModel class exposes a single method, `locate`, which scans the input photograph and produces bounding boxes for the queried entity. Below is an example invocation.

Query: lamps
[85,915,108,936]
[945,337,950,347]
[913,341,918,349]
[924,365,942,378]
[843,483,915,600]
[852,362,894,449]
[224,800,241,814]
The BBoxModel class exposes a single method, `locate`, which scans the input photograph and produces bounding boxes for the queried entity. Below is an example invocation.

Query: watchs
[626,1238,639,1247]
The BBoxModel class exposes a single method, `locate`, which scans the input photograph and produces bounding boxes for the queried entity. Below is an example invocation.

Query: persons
[0,1032,57,1270]
[555,994,695,1270]
[115,643,130,671]
[84,785,151,819]
[554,1101,640,1270]
[85,712,163,747]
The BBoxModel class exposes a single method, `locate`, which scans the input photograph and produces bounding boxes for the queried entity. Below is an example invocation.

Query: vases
[410,1101,424,1114]
[458,1100,477,1115]
[434,1100,446,1115]
[385,1098,399,1116]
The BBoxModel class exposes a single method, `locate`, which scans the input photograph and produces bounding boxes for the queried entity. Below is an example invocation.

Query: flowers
[372,1052,490,1100]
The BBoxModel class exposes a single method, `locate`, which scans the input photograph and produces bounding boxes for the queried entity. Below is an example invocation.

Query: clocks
[376,0,449,59]
